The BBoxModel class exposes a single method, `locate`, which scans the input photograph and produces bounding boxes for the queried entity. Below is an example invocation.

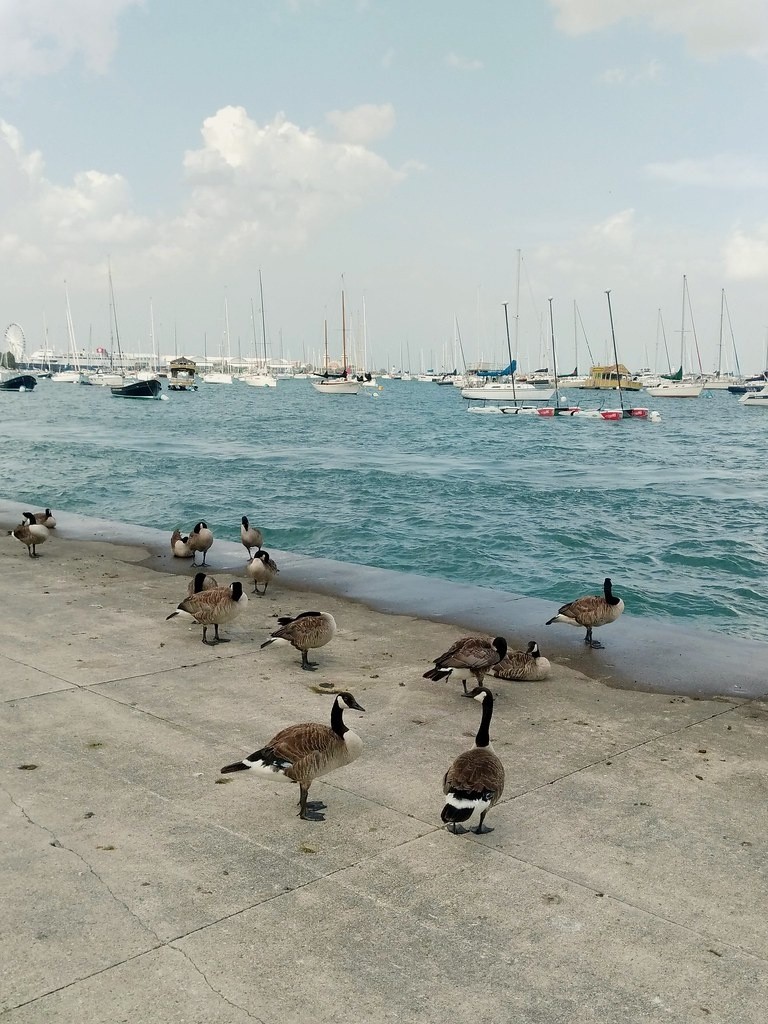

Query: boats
[0,250,379,388]
[0,374,38,392]
[381,247,768,427]
[310,271,366,395]
[109,375,162,401]
[245,269,279,386]
[166,350,199,391]
[199,296,234,384]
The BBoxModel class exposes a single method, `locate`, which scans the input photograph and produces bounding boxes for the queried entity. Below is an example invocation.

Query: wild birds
[441,686,505,835]
[544,578,625,649]
[221,693,367,823]
[486,641,551,682]
[8,508,57,559]
[260,611,336,672]
[166,514,280,646]
[422,636,507,702]
